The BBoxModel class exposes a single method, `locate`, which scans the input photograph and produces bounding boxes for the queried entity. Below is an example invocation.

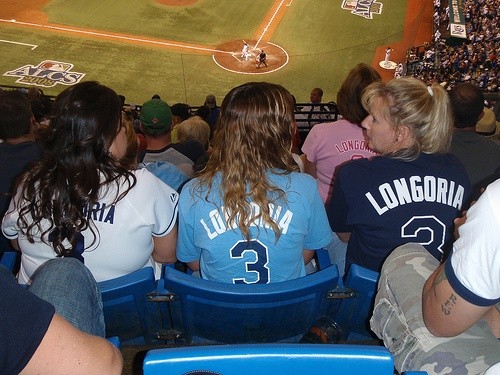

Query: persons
[370,178,500,375]
[0,258,123,375]
[324,77,470,273]
[0,0,500,201]
[1,81,177,284]
[176,82,332,285]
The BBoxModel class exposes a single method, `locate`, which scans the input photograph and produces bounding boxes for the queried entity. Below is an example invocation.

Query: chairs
[99,245,427,375]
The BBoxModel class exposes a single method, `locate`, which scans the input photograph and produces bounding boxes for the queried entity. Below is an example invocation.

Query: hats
[140,99,172,135]
[206,95,215,108]
[475,107,500,136]
